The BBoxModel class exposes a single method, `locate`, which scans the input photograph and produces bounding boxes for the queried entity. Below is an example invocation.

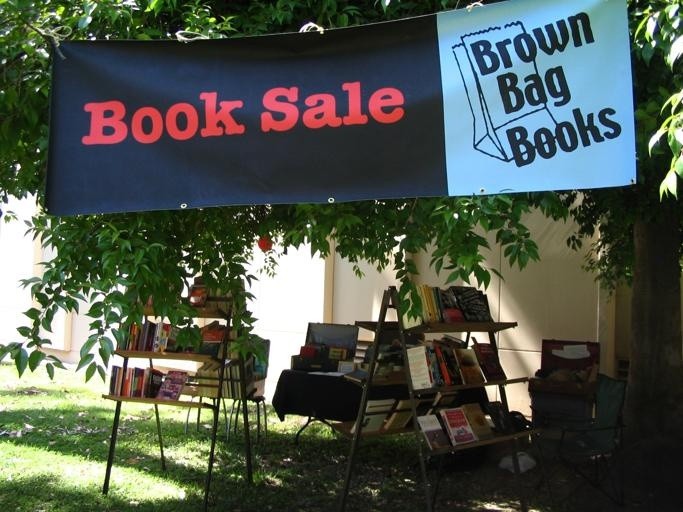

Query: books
[109,276,270,400]
[343,285,507,451]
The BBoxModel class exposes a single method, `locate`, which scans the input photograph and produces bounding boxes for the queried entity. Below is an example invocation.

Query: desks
[272,371,363,447]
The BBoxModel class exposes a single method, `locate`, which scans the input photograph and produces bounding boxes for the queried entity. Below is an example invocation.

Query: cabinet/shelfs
[100,276,270,512]
[337,286,540,512]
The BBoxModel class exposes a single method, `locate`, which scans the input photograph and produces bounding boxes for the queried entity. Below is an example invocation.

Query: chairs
[532,373,631,509]
